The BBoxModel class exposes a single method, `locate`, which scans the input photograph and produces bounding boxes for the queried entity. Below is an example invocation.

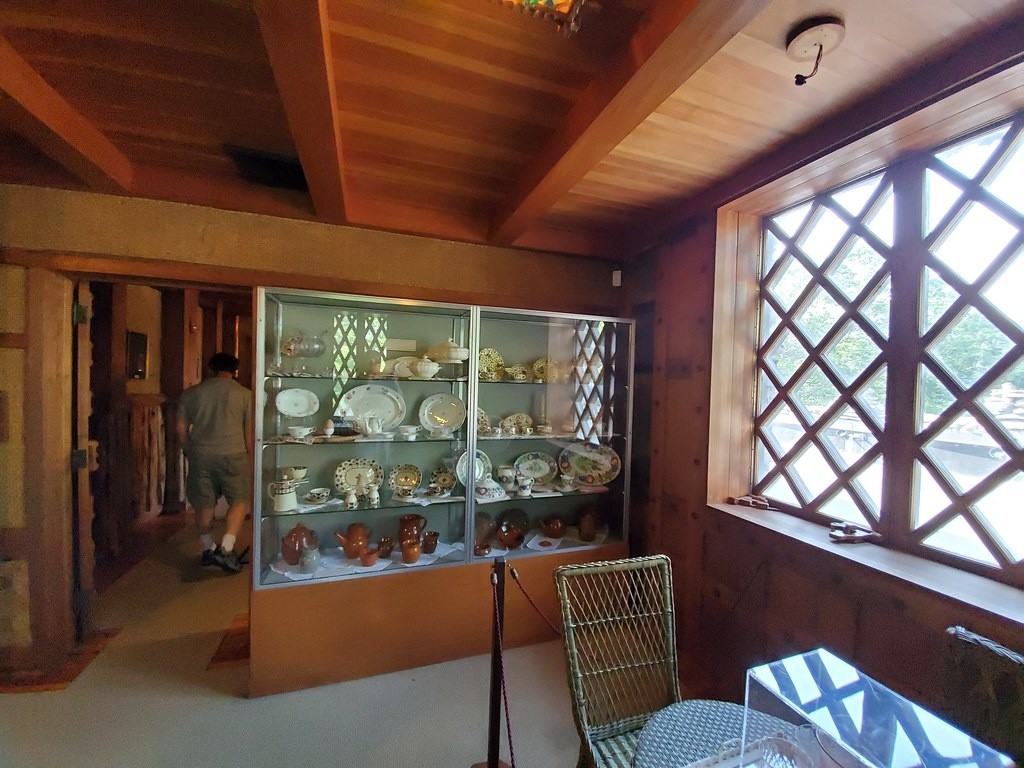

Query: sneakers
[201,545,243,572]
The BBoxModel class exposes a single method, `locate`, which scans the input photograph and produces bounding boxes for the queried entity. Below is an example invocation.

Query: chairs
[552,555,682,768]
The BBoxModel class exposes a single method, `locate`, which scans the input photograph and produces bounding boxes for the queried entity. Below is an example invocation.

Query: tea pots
[362,413,385,439]
[268,475,297,512]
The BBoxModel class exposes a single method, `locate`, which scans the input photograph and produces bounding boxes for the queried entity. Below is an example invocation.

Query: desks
[632,699,814,767]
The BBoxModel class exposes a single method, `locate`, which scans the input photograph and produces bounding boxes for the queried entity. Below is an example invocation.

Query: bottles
[422,532,439,554]
[474,511,497,544]
[578,513,597,541]
[497,465,516,489]
[281,523,321,574]
[496,523,526,549]
[345,488,359,511]
[401,539,421,563]
[367,485,381,506]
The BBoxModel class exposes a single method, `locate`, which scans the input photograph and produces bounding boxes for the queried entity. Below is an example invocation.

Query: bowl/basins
[405,337,469,379]
[474,543,491,556]
[285,466,307,480]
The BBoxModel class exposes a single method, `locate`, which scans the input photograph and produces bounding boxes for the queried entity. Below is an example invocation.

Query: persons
[178,354,254,573]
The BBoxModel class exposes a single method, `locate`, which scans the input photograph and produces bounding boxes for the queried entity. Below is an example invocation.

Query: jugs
[334,522,373,559]
[539,516,567,539]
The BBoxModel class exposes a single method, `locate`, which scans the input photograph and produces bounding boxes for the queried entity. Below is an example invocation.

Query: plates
[558,441,622,486]
[388,463,423,494]
[456,449,493,487]
[429,467,458,494]
[338,384,407,434]
[334,458,384,495]
[479,348,564,383]
[555,485,578,492]
[476,408,532,437]
[419,392,467,432]
[513,451,559,486]
[275,388,319,418]
[390,356,420,378]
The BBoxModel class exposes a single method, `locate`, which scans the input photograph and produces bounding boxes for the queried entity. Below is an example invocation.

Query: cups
[491,424,552,437]
[399,425,422,439]
[398,514,427,544]
[561,474,574,486]
[432,425,449,438]
[288,426,312,439]
[512,373,526,380]
[359,536,394,567]
[308,487,330,504]
[516,476,535,497]
[479,372,497,381]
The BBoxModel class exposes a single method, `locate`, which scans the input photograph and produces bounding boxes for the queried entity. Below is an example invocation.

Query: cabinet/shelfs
[246,285,637,699]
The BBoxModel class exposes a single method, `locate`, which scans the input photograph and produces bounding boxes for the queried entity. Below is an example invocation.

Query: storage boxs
[739,647,1016,767]
